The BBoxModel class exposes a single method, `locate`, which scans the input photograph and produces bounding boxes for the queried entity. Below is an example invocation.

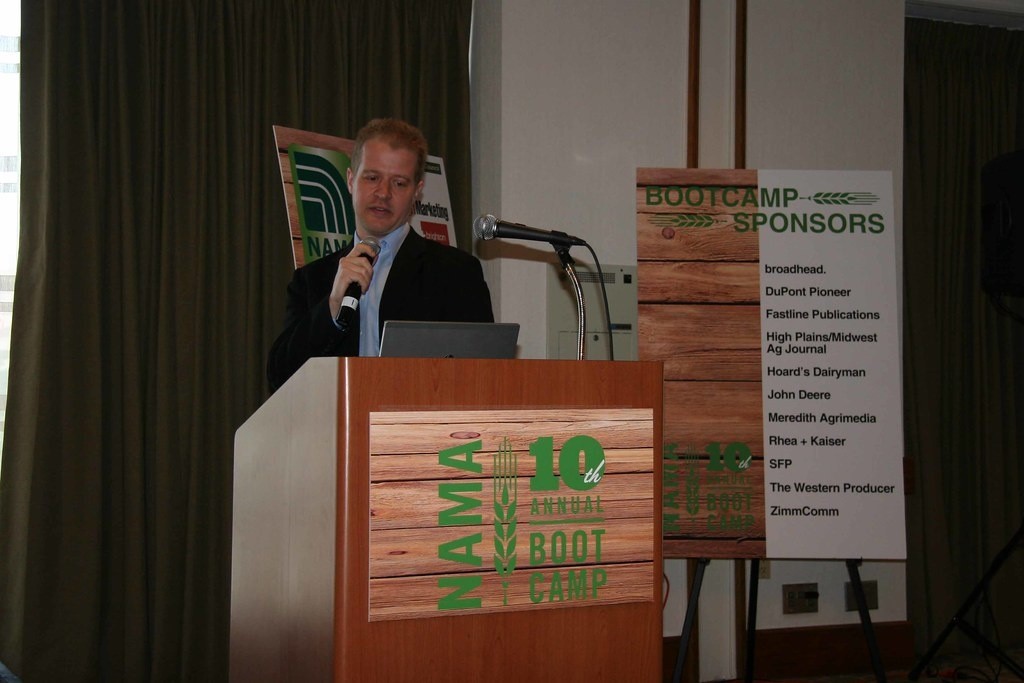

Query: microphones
[473,214,586,246]
[336,237,382,327]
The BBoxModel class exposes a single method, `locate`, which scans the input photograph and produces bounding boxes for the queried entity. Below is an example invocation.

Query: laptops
[379,321,520,359]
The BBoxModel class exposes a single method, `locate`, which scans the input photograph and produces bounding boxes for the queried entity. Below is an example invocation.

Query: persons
[266,117,495,397]
